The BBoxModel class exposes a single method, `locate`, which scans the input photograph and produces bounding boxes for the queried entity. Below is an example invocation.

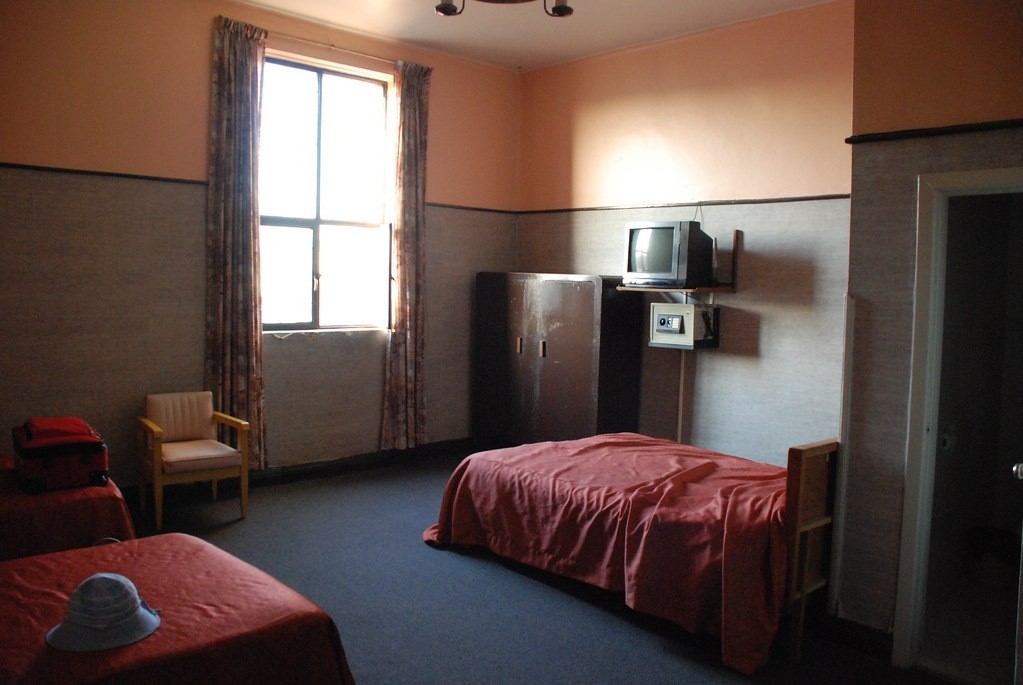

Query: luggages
[13,417,110,491]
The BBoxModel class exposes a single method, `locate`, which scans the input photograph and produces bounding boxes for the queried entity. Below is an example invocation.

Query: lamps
[435,0,573,17]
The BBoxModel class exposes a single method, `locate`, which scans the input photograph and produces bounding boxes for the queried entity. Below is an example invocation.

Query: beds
[0,533,356,685]
[422,432,838,673]
[0,455,136,559]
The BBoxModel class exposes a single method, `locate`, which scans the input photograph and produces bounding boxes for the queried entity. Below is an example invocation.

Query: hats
[46,572,161,651]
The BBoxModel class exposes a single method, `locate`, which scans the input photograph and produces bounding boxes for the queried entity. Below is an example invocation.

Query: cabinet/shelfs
[471,271,643,449]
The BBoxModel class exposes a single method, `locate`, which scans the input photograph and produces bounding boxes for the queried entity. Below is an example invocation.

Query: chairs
[134,391,249,532]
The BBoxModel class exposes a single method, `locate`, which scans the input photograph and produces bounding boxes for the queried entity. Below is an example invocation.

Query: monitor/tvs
[621,221,714,289]
